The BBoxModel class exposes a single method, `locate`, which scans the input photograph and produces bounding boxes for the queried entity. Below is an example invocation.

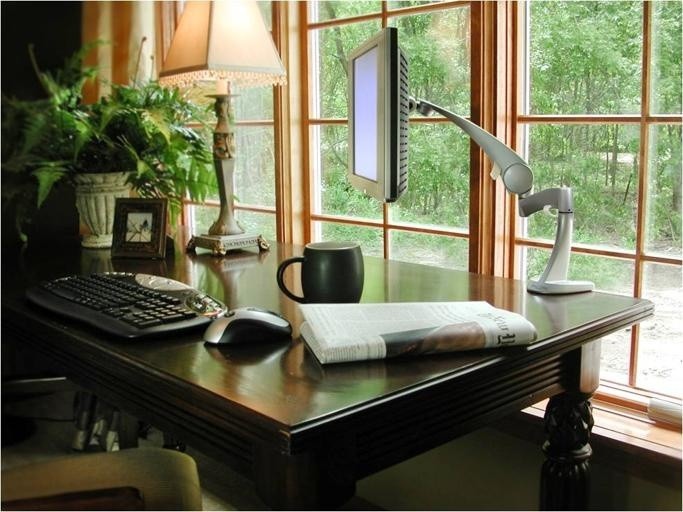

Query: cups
[276,241,364,303]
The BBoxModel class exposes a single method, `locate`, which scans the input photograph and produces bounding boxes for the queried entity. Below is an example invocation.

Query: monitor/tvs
[347,25,411,203]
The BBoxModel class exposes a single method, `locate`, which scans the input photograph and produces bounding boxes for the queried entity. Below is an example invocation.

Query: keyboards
[23,270,230,341]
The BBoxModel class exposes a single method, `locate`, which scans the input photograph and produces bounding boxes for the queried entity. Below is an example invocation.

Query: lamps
[160,1,288,255]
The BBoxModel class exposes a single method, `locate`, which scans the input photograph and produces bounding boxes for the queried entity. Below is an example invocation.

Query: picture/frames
[110,196,168,263]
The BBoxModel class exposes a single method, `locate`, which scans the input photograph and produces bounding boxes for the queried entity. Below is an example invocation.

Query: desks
[2,243,655,511]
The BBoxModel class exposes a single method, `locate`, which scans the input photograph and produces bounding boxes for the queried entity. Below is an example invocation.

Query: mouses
[202,305,294,346]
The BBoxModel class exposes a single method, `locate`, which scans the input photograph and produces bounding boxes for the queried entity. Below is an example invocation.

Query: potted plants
[0,36,241,253]
